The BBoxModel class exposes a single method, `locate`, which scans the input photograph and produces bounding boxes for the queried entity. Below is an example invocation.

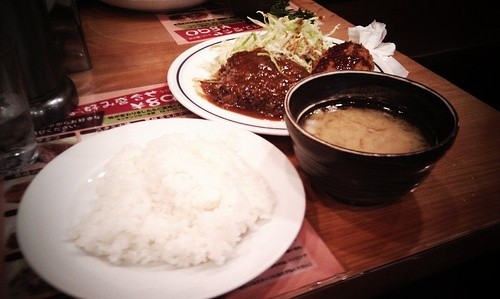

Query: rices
[60,132,274,269]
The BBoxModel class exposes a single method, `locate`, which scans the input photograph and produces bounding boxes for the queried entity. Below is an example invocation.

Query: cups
[0,44,40,176]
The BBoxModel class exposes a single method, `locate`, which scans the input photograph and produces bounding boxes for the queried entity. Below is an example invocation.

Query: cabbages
[218,11,342,75]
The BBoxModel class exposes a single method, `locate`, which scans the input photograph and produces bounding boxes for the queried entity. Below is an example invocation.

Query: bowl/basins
[284,70,459,207]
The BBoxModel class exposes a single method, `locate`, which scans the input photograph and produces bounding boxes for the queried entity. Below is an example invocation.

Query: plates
[17,118,307,298]
[167,31,385,135]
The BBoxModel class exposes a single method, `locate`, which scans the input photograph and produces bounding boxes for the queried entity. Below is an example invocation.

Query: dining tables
[1,0,499,298]
